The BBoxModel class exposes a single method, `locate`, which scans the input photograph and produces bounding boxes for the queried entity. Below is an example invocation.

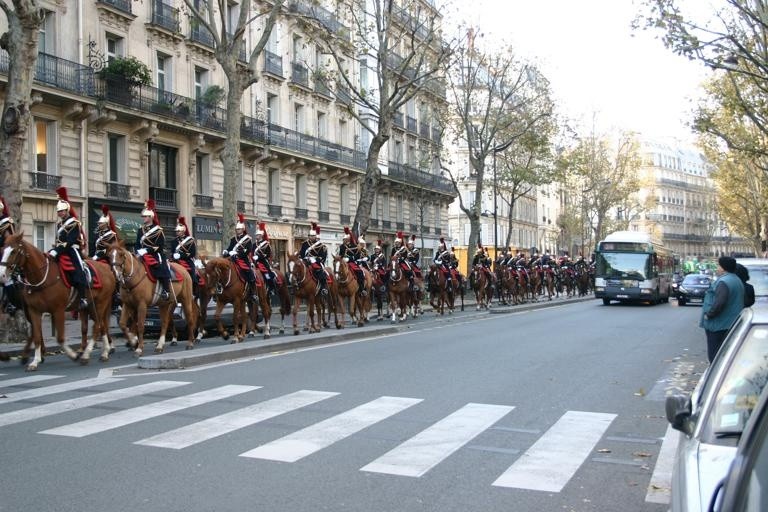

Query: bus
[594,229,680,307]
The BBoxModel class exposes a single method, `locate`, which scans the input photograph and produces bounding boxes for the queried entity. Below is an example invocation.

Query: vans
[117,259,265,337]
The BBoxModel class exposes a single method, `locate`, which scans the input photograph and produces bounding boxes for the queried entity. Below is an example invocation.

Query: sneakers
[320,283,465,296]
[81,289,276,308]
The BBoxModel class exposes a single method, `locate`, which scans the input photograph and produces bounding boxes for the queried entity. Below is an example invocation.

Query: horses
[0,230,595,373]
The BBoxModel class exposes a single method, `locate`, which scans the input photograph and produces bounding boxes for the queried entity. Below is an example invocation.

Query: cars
[664,301,768,511]
[672,274,682,297]
[709,383,768,512]
[676,274,713,306]
[732,257,768,302]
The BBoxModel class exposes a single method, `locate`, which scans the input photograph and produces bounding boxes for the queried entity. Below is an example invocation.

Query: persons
[170,231,198,288]
[0,202,18,302]
[697,256,746,362]
[135,216,171,300]
[730,262,755,307]
[45,209,91,312]
[91,221,123,310]
[223,215,421,316]
[430,240,599,301]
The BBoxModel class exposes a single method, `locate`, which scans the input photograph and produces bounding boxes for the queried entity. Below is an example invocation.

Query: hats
[57,187,265,236]
[719,257,735,272]
[476,245,584,262]
[309,222,455,258]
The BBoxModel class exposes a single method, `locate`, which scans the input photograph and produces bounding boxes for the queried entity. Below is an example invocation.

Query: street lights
[580,177,613,292]
[494,138,519,300]
